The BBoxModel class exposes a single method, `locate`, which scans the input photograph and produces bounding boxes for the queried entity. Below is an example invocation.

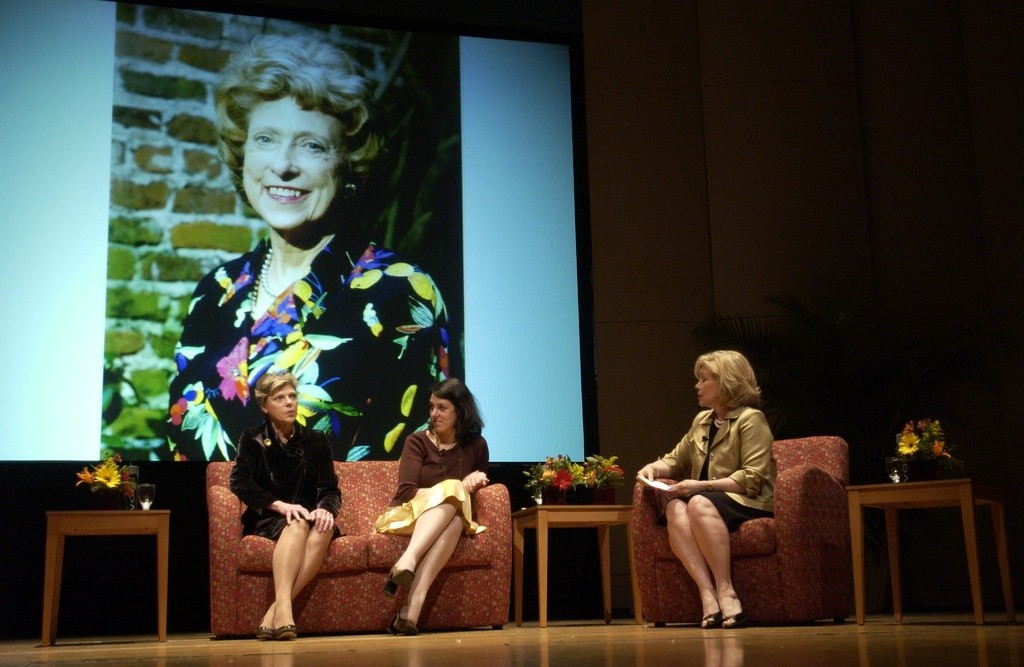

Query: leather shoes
[275,625,296,640]
[257,627,275,640]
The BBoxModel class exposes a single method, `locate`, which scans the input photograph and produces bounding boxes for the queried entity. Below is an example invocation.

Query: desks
[511,505,643,628]
[845,478,1016,625]
[41,510,171,647]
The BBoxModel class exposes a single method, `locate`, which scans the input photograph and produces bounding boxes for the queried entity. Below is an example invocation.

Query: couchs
[633,436,849,627]
[205,460,512,639]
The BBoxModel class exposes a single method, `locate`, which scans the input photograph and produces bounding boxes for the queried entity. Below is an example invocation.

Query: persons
[228,373,347,640]
[162,33,451,462]
[368,377,490,636]
[636,350,776,629]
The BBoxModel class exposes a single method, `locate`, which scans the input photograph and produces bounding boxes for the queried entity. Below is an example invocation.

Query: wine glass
[136,483,156,511]
[885,455,903,484]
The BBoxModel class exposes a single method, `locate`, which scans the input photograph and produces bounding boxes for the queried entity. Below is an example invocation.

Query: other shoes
[701,611,721,628]
[721,603,747,628]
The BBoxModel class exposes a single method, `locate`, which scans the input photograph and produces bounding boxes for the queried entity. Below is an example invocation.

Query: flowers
[888,418,966,469]
[76,454,137,499]
[523,455,626,499]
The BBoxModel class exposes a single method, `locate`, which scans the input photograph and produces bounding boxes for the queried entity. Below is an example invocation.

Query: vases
[588,487,615,504]
[541,487,566,505]
[95,490,121,510]
[911,460,942,481]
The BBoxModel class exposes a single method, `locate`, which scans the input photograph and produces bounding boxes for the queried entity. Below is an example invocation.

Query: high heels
[387,565,415,593]
[387,607,418,636]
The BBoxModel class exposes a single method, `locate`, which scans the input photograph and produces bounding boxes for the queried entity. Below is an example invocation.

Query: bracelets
[706,479,713,491]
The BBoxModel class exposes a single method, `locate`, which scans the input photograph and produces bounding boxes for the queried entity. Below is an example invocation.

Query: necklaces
[250,248,280,318]
[436,437,464,481]
[714,419,724,428]
